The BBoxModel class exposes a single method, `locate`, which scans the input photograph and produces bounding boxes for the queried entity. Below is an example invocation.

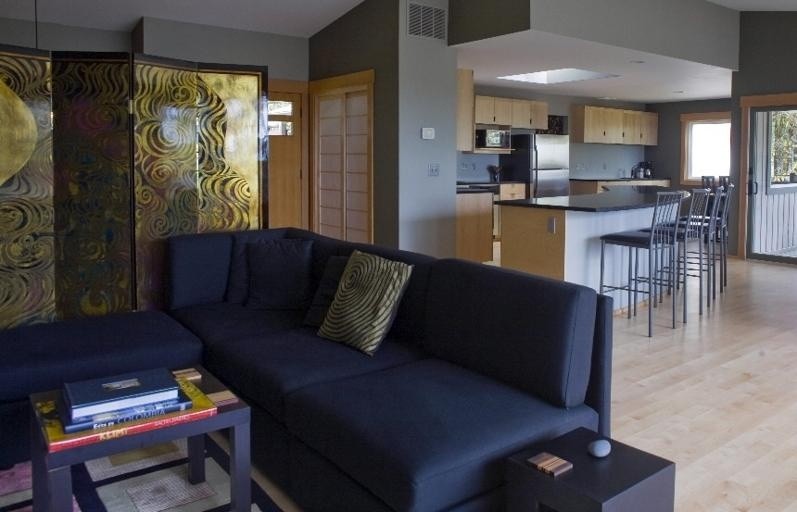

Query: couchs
[0,227,613,511]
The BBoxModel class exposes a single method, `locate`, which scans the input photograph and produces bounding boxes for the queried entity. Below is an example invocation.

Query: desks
[505,425,675,512]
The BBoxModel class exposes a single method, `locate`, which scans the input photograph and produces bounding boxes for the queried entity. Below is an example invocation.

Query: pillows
[316,249,414,356]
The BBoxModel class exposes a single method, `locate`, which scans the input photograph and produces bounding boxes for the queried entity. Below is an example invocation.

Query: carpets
[0,387,396,512]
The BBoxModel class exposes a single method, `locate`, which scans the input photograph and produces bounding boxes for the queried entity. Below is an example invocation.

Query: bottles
[635,160,652,179]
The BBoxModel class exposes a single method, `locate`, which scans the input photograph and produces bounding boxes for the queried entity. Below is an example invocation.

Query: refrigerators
[498,127,570,199]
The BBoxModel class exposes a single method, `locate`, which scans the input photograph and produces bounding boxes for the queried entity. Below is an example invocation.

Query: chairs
[598,175,735,338]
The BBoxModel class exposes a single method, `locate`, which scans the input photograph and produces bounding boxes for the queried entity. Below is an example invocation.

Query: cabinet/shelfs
[455,184,525,264]
[569,104,658,146]
[456,68,475,152]
[569,180,671,196]
[474,95,549,130]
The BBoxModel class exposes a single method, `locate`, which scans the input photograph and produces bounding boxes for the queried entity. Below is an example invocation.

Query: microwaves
[475,128,507,148]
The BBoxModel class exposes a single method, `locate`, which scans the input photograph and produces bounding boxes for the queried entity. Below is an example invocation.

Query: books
[32,365,218,453]
